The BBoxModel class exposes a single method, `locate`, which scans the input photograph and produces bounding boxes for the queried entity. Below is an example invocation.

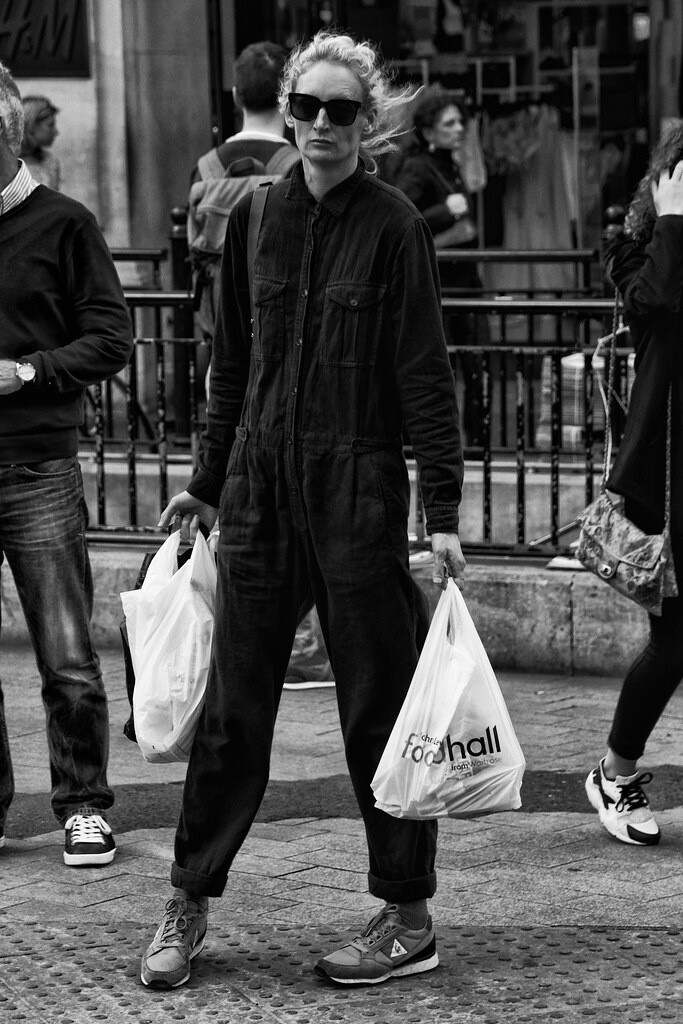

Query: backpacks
[186,145,300,255]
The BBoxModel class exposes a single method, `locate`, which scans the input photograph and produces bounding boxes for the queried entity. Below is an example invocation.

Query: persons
[142,34,467,988]
[0,64,133,870]
[397,94,488,460]
[189,42,302,404]
[20,96,60,192]
[584,116,683,846]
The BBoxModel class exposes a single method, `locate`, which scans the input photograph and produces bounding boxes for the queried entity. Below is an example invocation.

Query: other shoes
[281,650,336,691]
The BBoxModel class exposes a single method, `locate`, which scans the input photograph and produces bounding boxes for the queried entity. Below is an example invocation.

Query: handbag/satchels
[370,577,527,820]
[120,521,216,744]
[402,160,477,248]
[575,494,678,617]
[119,529,218,765]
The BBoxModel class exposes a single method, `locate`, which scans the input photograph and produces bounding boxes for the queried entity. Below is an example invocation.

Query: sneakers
[63,814,116,865]
[141,889,207,989]
[314,904,439,984]
[585,756,662,847]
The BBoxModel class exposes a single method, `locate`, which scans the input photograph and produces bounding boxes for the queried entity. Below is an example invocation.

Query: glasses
[289,92,370,128]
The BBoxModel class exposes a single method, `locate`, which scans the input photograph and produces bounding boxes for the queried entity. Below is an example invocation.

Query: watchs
[15,360,35,385]
